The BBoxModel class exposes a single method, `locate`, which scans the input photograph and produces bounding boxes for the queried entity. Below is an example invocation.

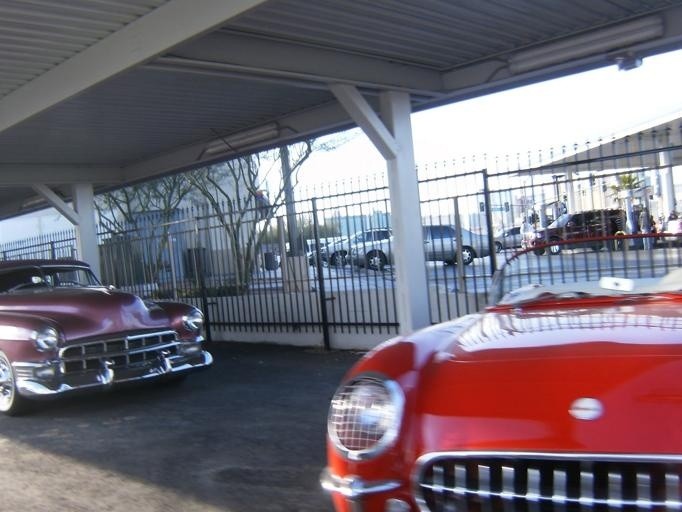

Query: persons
[666,209,677,220]
[639,207,651,250]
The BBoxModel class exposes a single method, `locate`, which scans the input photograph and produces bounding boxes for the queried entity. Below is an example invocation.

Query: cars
[0,259,214,414]
[318,232,682,511]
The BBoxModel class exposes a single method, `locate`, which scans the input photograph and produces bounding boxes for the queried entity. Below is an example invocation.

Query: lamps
[204,121,280,156]
[21,194,46,209]
[508,13,665,75]
